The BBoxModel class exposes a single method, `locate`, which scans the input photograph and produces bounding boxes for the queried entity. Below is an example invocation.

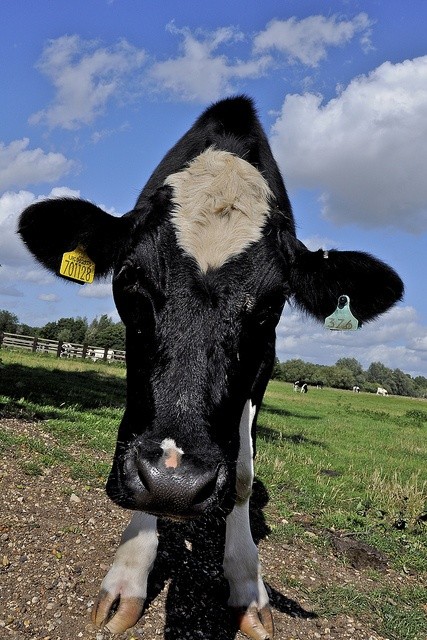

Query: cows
[317,380,324,388]
[376,387,389,397]
[352,386,360,392]
[17,94,405,640]
[294,380,307,393]
